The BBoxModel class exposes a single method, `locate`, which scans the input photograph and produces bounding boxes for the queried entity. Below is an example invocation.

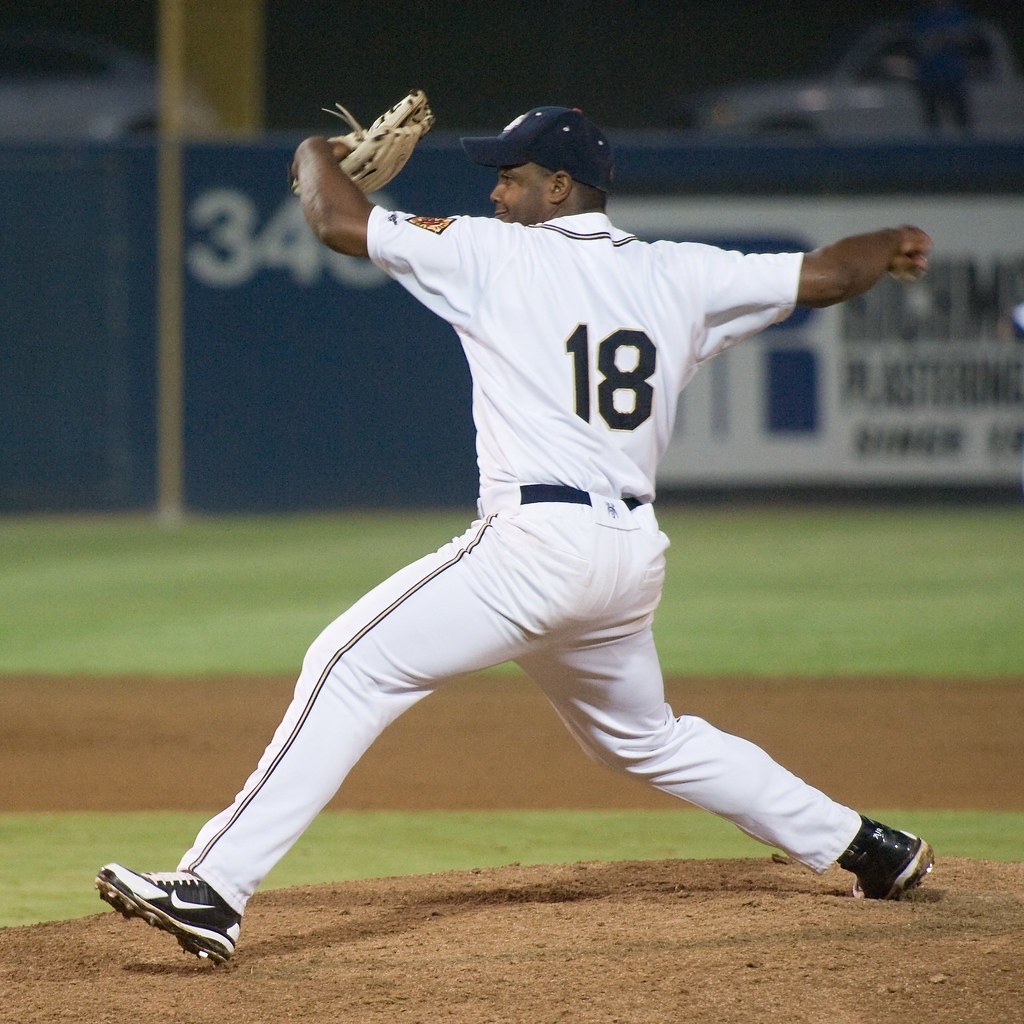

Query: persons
[95,109,933,967]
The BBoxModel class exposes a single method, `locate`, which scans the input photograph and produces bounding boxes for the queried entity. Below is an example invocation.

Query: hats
[463,106,613,192]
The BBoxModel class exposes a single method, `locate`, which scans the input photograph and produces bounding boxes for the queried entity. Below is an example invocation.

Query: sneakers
[839,815,936,900]
[94,862,240,965]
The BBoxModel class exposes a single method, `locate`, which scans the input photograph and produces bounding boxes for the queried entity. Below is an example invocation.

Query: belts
[521,483,645,513]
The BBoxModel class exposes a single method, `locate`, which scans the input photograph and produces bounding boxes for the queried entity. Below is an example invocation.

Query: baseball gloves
[285,90,435,200]
